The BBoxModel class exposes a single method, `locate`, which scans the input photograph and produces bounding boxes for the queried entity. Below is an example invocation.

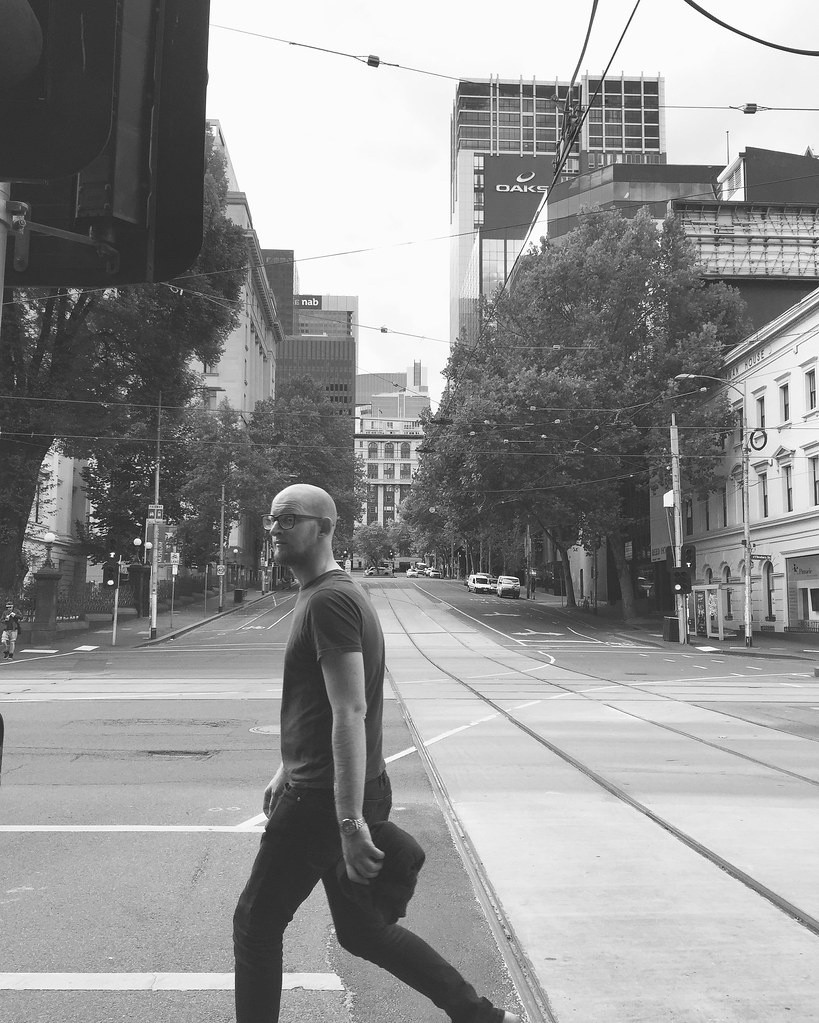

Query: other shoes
[8,653,13,660]
[3,651,9,660]
[503,1010,520,1023]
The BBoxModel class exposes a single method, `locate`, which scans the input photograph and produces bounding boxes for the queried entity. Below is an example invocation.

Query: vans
[497,576,521,599]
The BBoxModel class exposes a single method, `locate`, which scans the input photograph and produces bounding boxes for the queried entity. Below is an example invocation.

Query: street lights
[677,370,754,650]
[149,387,228,640]
[106,578,119,646]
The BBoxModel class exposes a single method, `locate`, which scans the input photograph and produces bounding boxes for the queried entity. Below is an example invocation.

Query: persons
[233,484,521,1023]
[1,602,23,661]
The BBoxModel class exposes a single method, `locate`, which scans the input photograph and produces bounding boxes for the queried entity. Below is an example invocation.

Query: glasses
[261,514,321,531]
[6,607,12,609]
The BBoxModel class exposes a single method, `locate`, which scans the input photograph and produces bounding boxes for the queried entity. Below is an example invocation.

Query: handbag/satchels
[17,623,21,635]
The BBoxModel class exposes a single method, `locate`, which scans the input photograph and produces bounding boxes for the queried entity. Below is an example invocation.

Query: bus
[415,562,427,574]
[379,561,394,575]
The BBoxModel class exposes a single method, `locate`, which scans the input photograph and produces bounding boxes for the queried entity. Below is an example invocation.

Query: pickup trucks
[407,568,418,578]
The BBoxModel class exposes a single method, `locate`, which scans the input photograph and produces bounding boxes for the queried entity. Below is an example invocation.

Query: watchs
[338,818,365,834]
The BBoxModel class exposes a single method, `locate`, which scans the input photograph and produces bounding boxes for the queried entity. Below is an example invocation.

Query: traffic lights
[673,568,692,594]
[680,544,697,582]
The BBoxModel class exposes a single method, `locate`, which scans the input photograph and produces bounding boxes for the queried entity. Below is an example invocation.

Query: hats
[6,601,13,607]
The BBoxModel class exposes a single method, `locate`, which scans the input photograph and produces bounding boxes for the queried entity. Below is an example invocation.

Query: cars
[365,566,386,576]
[488,578,498,594]
[476,573,493,579]
[525,567,538,575]
[467,575,490,594]
[422,567,441,578]
